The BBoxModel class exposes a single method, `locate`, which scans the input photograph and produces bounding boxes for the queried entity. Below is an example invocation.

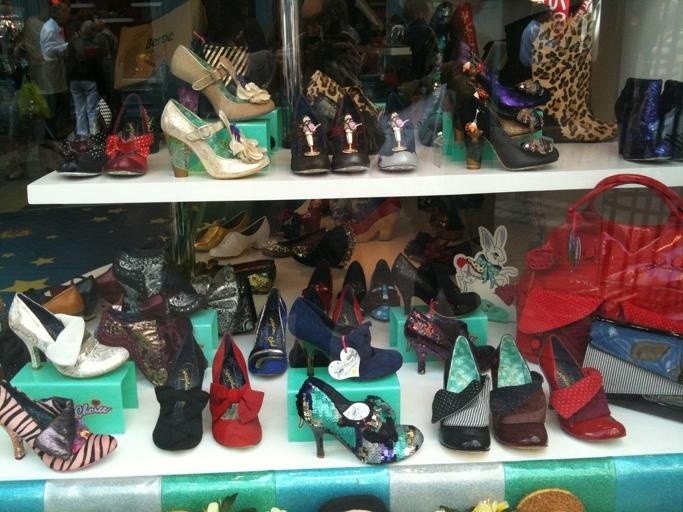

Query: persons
[64,19,102,136]
[70,11,116,110]
[40,2,69,147]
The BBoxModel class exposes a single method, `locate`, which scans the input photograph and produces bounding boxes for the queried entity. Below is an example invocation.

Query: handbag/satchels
[580,314,683,411]
[516,173,682,363]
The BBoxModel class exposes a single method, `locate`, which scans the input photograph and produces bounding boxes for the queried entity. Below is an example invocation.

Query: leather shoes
[71,275,97,321]
[193,209,249,252]
[209,213,270,259]
[307,259,332,313]
[41,286,85,319]
[368,259,402,322]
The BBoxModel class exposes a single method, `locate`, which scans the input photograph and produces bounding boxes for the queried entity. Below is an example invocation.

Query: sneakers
[263,225,327,257]
[198,257,274,295]
[290,225,354,269]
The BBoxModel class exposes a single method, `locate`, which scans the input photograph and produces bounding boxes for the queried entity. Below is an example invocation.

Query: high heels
[333,284,362,326]
[168,44,275,123]
[152,331,211,451]
[209,331,264,449]
[445,65,545,140]
[288,297,403,383]
[0,379,118,473]
[7,292,129,379]
[489,333,549,450]
[403,308,497,375]
[331,93,369,173]
[453,96,559,169]
[350,197,401,242]
[105,93,154,177]
[392,253,481,316]
[379,91,418,171]
[296,378,424,466]
[538,332,627,440]
[56,96,113,176]
[404,230,482,264]
[160,97,271,178]
[248,286,288,378]
[431,334,491,452]
[279,199,329,228]
[290,93,330,172]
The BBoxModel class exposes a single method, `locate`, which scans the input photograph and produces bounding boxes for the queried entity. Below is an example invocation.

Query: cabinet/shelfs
[0,0,683,511]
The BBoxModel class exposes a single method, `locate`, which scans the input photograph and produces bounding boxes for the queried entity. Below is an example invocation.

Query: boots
[533,0,619,143]
[615,78,671,161]
[430,198,463,239]
